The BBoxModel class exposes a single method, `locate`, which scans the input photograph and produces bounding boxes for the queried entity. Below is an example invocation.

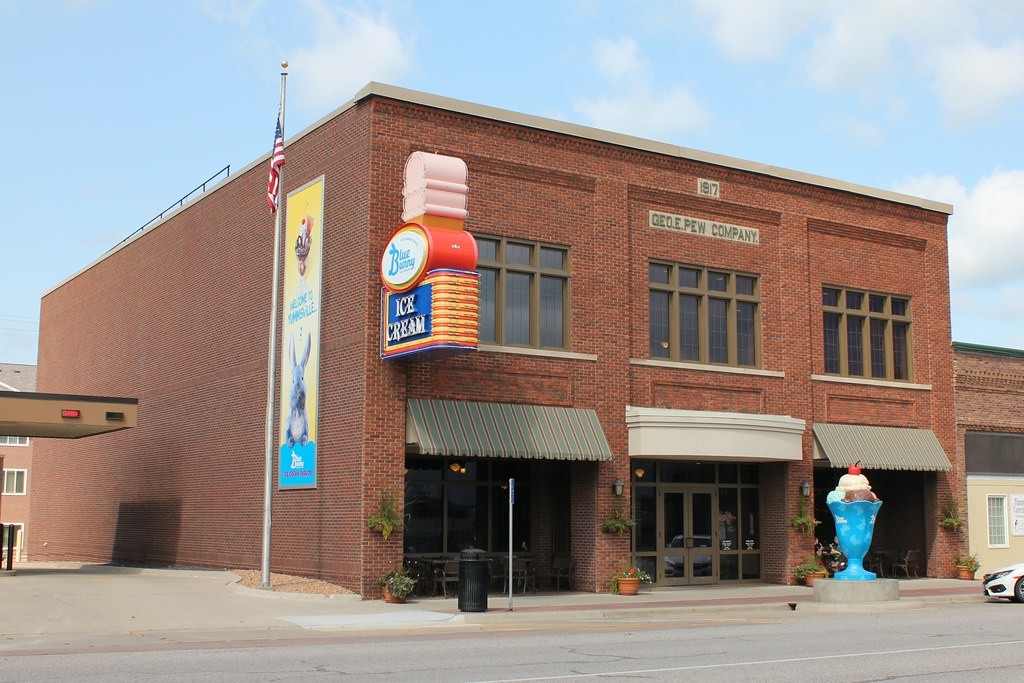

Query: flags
[265,75,287,215]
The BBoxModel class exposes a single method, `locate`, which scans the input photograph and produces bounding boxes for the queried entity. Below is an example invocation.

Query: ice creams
[827,473,880,503]
[295,202,314,276]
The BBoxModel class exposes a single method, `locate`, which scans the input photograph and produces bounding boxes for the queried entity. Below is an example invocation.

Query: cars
[982,561,1024,602]
[665,533,712,577]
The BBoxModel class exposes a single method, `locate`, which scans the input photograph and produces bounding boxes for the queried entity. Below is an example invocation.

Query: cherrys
[848,460,861,475]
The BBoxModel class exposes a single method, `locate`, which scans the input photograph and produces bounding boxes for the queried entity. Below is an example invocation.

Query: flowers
[795,556,828,582]
[609,561,652,596]
[378,571,418,597]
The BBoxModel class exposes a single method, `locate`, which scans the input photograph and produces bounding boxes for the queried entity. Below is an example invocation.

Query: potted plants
[366,478,422,541]
[953,554,980,581]
[792,506,823,534]
[939,505,964,531]
[602,506,637,536]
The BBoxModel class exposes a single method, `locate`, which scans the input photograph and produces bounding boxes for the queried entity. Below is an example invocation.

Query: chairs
[431,560,460,598]
[894,548,919,579]
[863,551,881,577]
[487,558,575,594]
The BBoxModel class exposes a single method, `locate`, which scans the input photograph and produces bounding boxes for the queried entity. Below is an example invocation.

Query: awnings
[812,423,952,472]
[404,398,613,462]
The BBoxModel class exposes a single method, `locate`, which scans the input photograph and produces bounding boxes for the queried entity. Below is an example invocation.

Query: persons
[814,537,844,573]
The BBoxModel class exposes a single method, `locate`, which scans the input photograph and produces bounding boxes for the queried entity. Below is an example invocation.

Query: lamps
[801,480,811,497]
[614,479,623,496]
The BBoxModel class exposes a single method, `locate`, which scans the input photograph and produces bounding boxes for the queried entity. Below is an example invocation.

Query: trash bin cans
[458,548,489,612]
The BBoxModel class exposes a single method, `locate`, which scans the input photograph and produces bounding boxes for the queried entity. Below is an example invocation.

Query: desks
[423,559,459,596]
[873,550,896,578]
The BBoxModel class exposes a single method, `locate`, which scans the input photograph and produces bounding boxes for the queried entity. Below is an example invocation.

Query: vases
[806,572,824,587]
[383,586,406,604]
[617,578,639,595]
